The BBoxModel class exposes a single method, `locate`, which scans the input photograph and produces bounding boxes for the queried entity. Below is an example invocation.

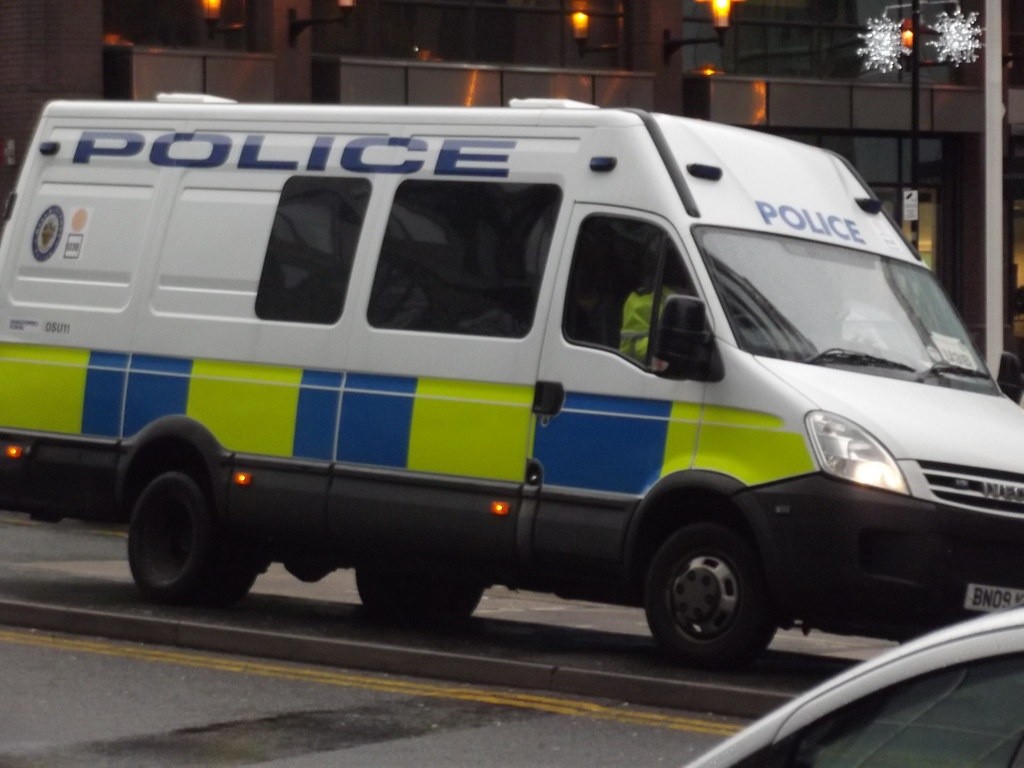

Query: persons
[617,261,688,373]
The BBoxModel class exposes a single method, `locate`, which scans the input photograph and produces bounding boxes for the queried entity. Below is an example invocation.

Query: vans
[1,90,1022,676]
